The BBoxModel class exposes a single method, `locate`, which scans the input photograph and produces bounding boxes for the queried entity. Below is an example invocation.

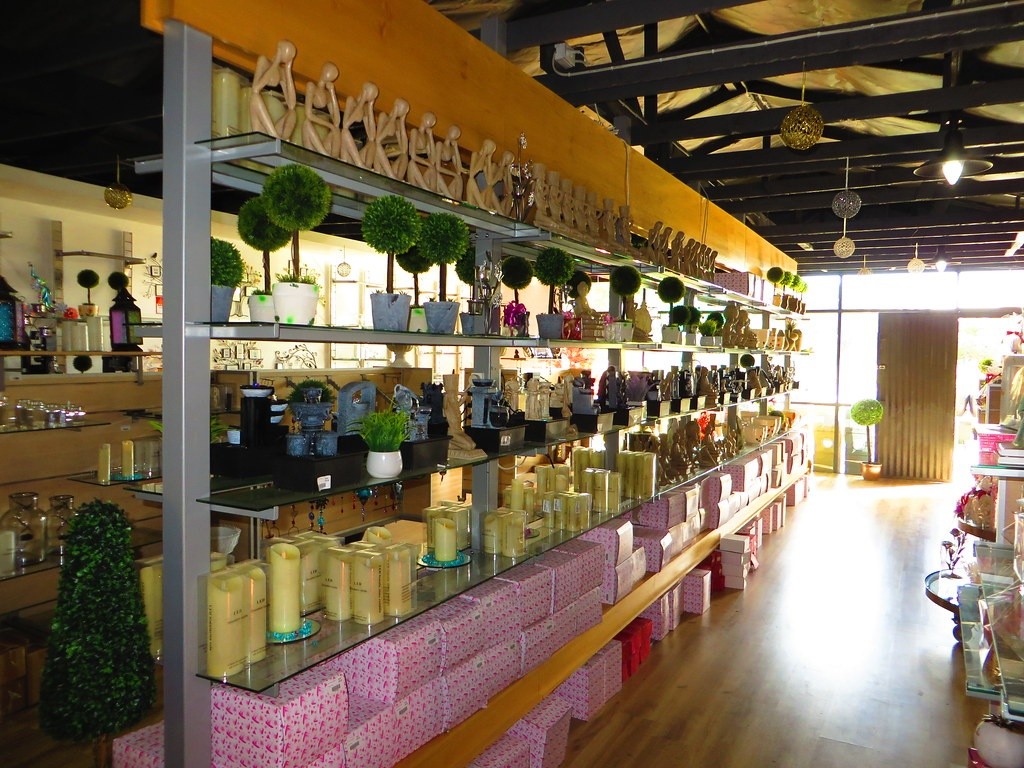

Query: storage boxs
[0,625,50,716]
[112,426,810,768]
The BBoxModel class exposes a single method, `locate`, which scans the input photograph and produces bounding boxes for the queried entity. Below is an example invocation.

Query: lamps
[912,115,993,186]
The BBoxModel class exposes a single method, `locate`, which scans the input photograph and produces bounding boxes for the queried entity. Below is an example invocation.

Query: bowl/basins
[211,525,241,554]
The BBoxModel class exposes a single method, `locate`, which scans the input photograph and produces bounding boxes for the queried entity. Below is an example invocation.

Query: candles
[100,439,658,679]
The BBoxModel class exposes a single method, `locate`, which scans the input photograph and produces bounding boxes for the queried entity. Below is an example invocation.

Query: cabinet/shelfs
[957,439,1024,722]
[139,0,811,768]
[0,408,243,581]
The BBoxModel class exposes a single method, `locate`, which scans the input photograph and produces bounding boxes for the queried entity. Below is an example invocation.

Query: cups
[61,317,103,352]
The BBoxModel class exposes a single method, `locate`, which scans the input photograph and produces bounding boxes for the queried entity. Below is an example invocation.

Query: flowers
[943,487,994,577]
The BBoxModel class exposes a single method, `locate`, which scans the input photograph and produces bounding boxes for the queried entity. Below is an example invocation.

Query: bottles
[0,492,80,578]
[0,394,86,424]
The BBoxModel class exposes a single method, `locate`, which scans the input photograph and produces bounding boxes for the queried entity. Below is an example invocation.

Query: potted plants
[209,164,807,478]
[77,269,100,321]
[851,399,884,481]
